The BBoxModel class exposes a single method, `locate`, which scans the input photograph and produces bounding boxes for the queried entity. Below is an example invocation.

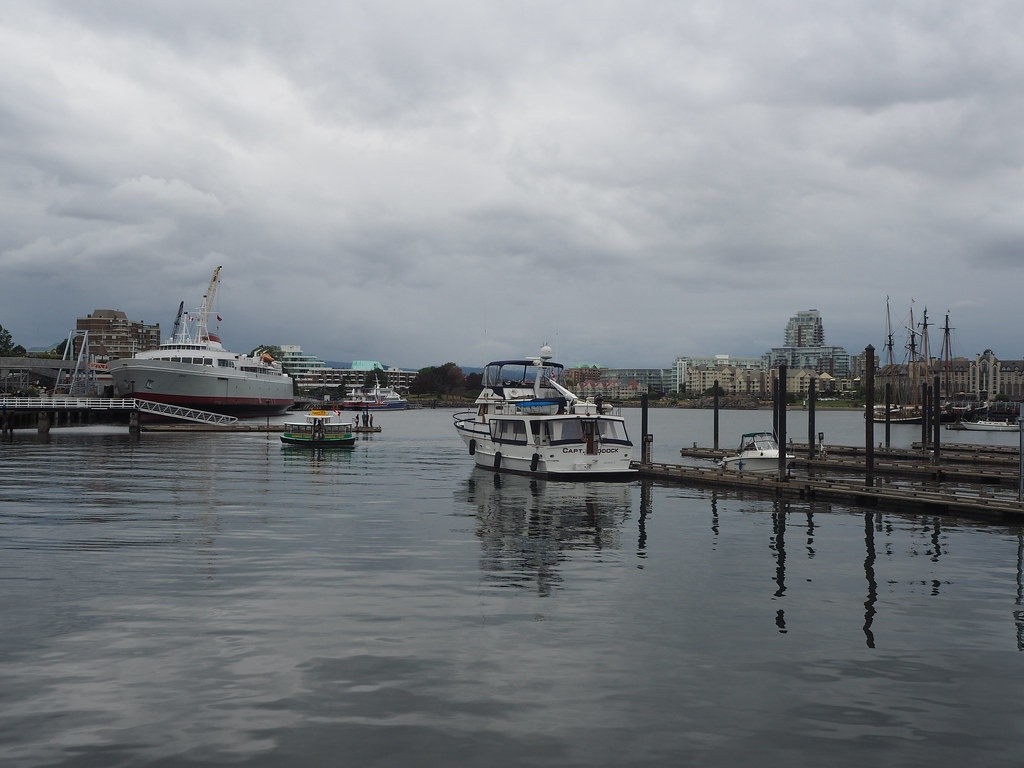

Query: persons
[355,414,359,427]
[362,411,373,427]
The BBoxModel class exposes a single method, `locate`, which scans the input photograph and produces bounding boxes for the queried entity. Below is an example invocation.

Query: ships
[107,266,295,419]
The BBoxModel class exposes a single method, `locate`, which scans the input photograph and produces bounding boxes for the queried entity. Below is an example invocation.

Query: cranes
[195,265,222,344]
[171,300,185,343]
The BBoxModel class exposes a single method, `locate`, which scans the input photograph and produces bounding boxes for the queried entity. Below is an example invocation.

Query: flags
[334,409,341,416]
[188,316,193,320]
[217,315,222,321]
[552,370,556,381]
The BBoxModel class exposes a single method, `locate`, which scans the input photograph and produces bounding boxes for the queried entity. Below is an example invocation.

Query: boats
[716,431,796,478]
[453,340,638,474]
[279,411,356,445]
[343,371,407,410]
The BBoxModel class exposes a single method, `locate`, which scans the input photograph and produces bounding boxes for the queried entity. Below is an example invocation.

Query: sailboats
[864,295,1021,431]
